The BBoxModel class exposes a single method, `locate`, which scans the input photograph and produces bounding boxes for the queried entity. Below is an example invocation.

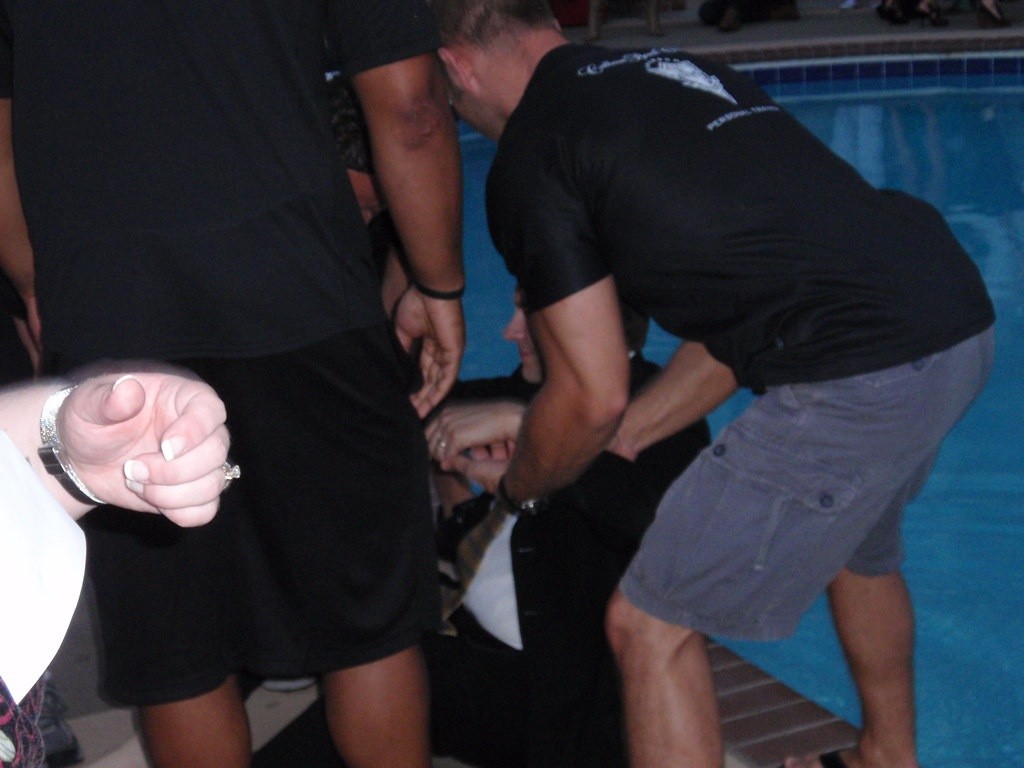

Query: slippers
[779,749,858,768]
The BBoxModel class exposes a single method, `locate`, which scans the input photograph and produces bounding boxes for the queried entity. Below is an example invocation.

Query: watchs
[496,476,543,514]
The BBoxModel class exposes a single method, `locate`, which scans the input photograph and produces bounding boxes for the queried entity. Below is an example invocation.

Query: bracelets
[414,281,464,299]
[37,384,108,506]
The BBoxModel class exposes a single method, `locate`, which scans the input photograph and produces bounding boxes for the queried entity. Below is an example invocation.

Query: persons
[427,0,998,768]
[0,0,465,768]
[1,270,84,768]
[251,68,712,766]
[0,370,230,768]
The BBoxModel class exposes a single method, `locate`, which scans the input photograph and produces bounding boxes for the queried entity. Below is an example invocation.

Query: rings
[223,462,241,489]
[437,440,447,448]
